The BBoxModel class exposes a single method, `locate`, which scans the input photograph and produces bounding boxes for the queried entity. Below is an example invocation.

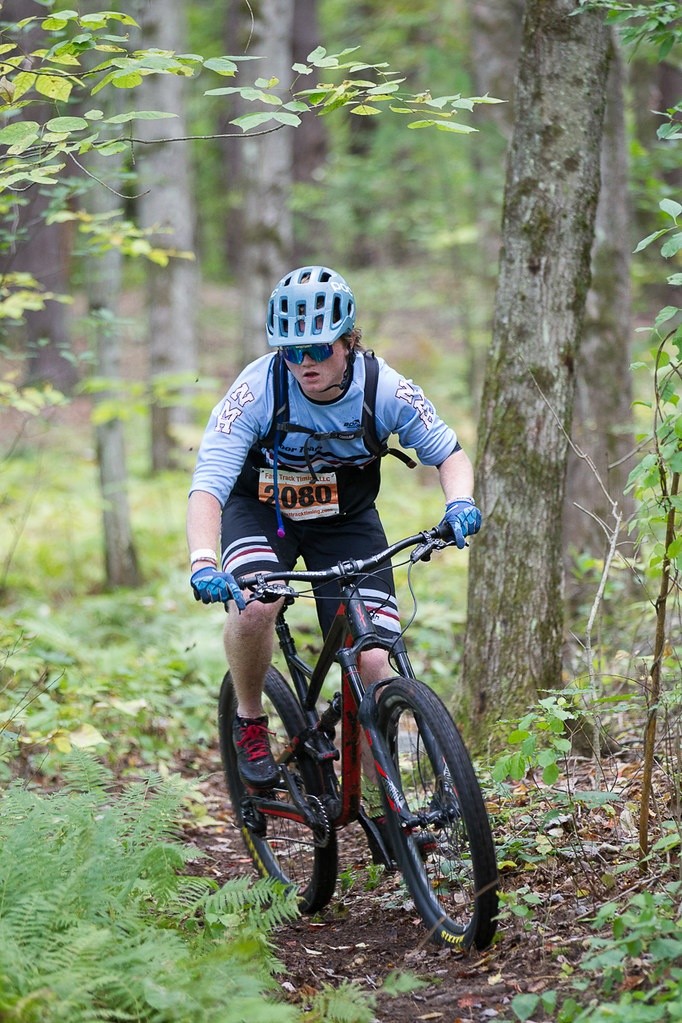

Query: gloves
[439,500,482,549]
[189,566,246,611]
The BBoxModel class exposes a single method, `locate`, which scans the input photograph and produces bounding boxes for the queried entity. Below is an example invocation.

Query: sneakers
[231,713,281,792]
[365,816,437,863]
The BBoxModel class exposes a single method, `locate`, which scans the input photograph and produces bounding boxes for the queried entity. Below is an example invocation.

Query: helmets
[264,266,356,345]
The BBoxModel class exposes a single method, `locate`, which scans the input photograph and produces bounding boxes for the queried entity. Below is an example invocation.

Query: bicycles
[217,517,502,955]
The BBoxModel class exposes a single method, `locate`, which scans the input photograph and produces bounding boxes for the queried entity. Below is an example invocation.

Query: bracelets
[190,549,218,572]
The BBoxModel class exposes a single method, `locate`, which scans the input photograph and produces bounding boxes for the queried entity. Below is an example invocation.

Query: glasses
[276,343,333,364]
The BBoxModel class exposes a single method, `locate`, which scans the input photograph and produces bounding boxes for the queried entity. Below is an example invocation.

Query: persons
[185,265,482,862]
